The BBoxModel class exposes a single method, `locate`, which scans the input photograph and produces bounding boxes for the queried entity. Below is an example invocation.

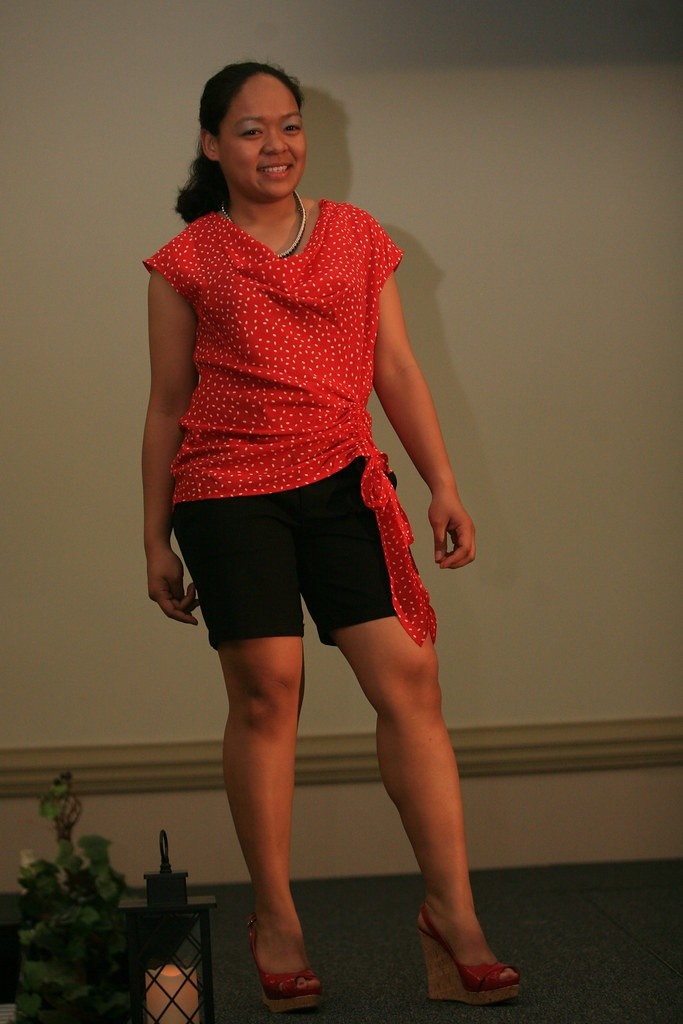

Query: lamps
[116,825,219,1024]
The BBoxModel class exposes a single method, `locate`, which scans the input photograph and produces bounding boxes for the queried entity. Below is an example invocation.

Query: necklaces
[221,189,309,260]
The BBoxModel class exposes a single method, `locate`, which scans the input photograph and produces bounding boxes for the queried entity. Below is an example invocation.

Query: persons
[145,60,524,1012]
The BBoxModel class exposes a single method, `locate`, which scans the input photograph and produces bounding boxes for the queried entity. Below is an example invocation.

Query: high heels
[416,900,521,1004]
[247,912,322,1012]
